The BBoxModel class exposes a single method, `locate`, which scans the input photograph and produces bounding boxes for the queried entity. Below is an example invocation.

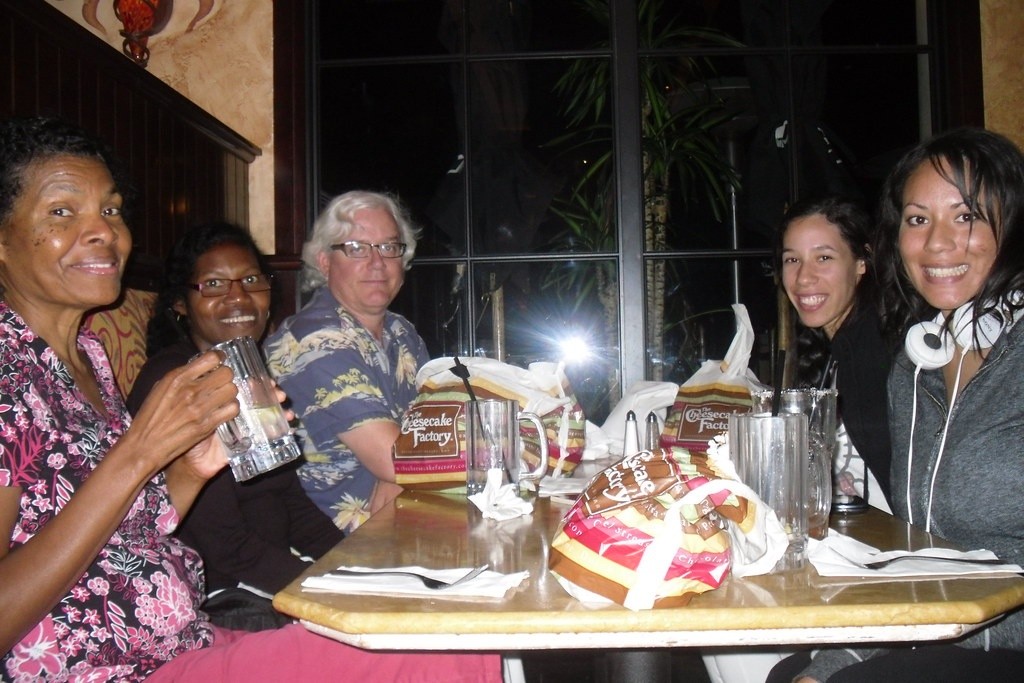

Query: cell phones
[829,495,867,514]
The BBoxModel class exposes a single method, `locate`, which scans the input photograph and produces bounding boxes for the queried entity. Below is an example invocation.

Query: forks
[328,564,490,592]
[829,546,1017,570]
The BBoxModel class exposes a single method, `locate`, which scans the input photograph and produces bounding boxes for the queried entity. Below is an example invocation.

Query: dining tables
[270,488,1023,683]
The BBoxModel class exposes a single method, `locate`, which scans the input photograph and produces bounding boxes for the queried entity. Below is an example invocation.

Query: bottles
[623,409,659,456]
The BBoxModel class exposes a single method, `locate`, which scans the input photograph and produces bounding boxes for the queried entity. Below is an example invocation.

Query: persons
[124,217,344,635]
[262,189,434,533]
[0,111,503,682]
[605,189,897,683]
[764,127,1024,683]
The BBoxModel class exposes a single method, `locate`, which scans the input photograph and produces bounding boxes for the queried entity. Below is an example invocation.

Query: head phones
[904,287,1024,370]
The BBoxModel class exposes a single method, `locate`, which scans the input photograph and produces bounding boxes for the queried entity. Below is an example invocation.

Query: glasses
[330,240,408,259]
[177,272,276,298]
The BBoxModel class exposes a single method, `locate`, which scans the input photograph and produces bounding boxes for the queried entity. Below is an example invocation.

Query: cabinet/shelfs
[1,0,264,293]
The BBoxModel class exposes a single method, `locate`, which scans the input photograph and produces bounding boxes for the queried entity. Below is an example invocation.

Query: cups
[464,400,549,502]
[779,389,837,541]
[187,334,301,482]
[727,413,832,572]
[749,381,773,415]
